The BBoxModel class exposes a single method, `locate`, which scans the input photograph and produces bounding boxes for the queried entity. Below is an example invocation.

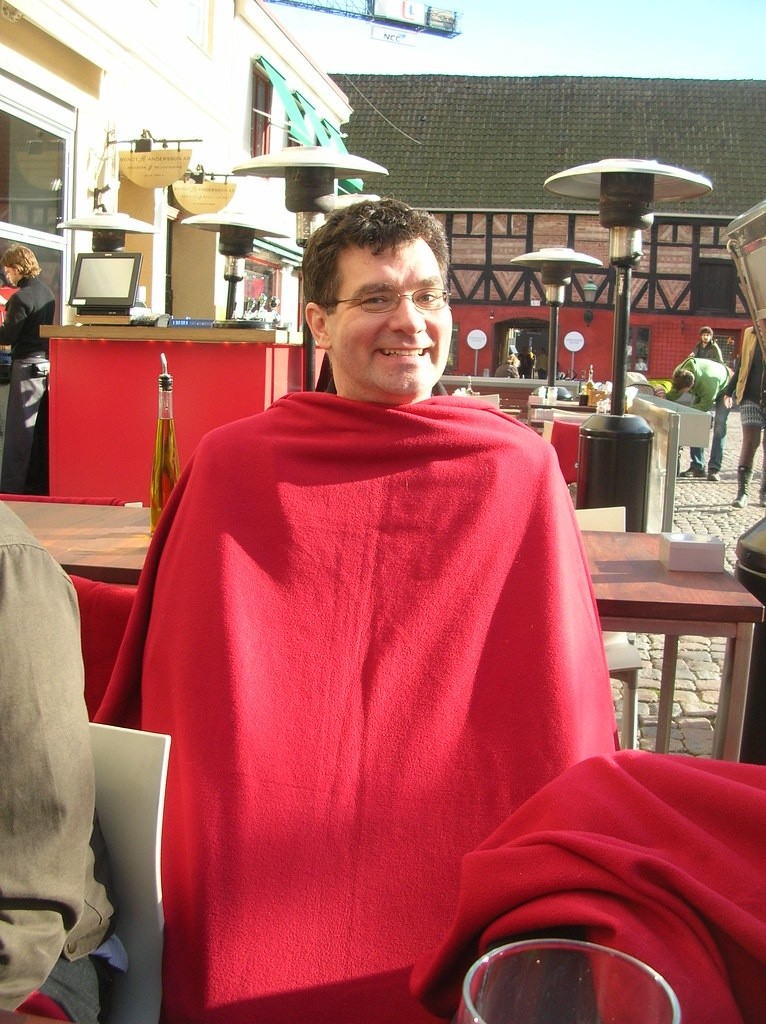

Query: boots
[732,465,754,508]
[758,468,766,507]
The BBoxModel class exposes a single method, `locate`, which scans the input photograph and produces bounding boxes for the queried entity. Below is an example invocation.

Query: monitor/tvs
[67,252,142,316]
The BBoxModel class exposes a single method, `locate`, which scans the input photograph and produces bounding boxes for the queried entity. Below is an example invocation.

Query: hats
[654,386,666,396]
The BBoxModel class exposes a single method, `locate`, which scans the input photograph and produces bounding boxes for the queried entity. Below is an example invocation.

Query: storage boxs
[660,531,725,572]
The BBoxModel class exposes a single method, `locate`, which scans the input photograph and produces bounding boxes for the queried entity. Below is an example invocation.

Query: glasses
[317,288,452,313]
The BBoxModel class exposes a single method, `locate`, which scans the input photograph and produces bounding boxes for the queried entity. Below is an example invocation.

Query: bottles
[586,364,595,406]
[149,353,180,537]
[538,386,547,399]
[547,387,559,401]
[466,375,473,397]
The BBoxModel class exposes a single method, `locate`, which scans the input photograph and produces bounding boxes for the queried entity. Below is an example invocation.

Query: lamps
[583,275,598,305]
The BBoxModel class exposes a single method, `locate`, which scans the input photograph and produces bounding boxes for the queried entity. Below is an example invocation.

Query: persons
[0,245,54,496]
[494,346,536,379]
[0,497,119,1024]
[689,327,723,363]
[654,357,735,481]
[303,199,454,410]
[723,326,766,508]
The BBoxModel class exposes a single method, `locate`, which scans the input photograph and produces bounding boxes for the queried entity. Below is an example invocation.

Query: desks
[529,399,597,484]
[2,501,765,764]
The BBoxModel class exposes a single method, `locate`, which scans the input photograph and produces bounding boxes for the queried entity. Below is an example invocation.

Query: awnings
[255,54,365,196]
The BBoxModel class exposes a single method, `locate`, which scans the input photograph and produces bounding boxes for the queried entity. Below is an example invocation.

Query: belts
[11,355,46,359]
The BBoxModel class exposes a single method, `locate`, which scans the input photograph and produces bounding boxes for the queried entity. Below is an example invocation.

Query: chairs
[575,506,646,749]
[89,722,170,1024]
[627,372,655,395]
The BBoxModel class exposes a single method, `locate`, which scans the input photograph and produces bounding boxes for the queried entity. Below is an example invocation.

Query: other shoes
[680,467,706,477]
[708,468,720,480]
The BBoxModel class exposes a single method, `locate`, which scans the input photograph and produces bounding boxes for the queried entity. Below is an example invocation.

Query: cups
[454,938,682,1024]
[578,394,588,406]
[472,392,480,396]
